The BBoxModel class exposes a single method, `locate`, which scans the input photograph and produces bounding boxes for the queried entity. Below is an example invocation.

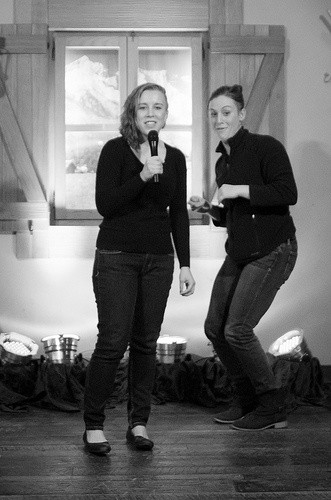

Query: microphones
[148,130,158,183]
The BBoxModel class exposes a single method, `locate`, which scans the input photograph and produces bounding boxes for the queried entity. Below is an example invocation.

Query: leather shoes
[83,430,111,454]
[126,424,154,450]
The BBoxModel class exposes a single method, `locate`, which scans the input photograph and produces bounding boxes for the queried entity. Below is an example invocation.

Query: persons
[189,84,298,431]
[81,82,195,456]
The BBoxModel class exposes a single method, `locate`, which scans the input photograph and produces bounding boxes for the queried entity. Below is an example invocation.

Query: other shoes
[214,406,256,423]
[231,410,289,431]
